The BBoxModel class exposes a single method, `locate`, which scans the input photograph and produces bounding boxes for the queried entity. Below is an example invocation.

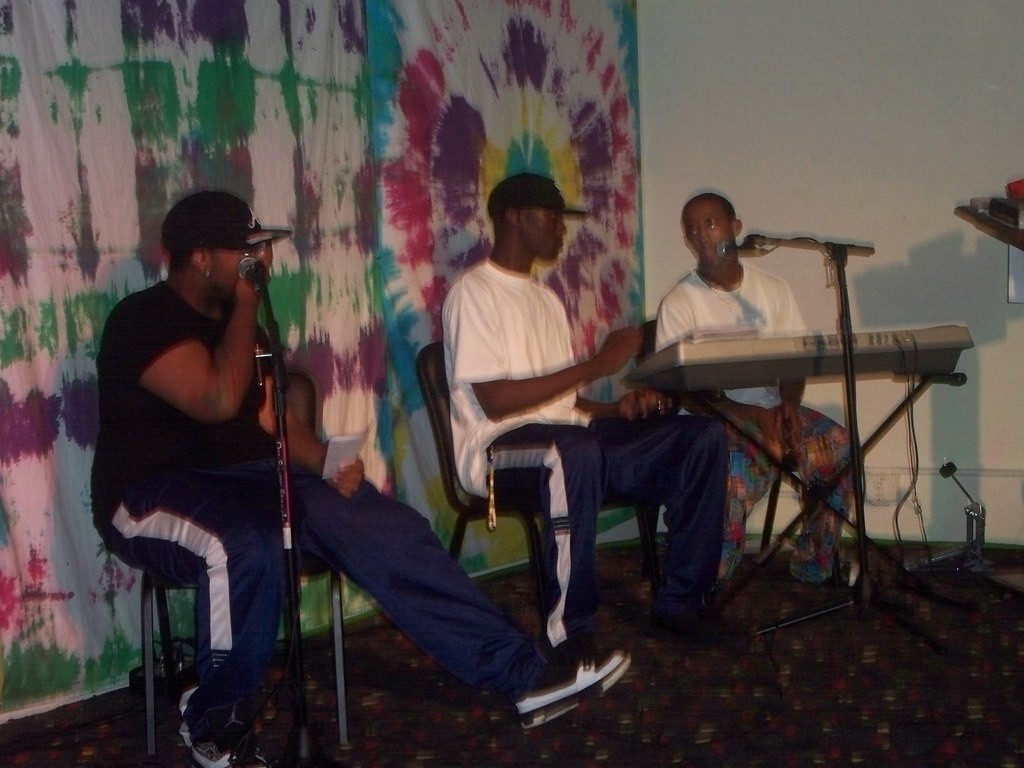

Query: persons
[654,192,864,593]
[88,190,634,768]
[440,171,731,653]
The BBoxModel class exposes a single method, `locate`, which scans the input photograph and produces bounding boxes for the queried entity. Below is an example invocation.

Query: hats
[161,191,293,251]
[489,173,587,217]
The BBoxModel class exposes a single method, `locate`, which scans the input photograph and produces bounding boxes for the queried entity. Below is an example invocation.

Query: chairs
[637,319,784,591]
[414,341,663,639]
[138,364,351,757]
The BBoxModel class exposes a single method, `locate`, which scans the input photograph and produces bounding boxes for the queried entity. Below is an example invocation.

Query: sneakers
[516,647,631,730]
[191,744,269,768]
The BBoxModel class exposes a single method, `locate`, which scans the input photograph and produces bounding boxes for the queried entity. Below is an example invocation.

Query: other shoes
[650,607,744,649]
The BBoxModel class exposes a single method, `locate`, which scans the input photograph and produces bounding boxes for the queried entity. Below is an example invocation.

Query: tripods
[741,234,951,655]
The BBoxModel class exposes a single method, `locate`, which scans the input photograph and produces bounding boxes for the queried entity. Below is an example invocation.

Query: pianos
[618,322,973,388]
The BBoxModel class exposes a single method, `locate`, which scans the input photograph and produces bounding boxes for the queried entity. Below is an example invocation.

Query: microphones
[238,258,263,294]
[717,241,770,257]
